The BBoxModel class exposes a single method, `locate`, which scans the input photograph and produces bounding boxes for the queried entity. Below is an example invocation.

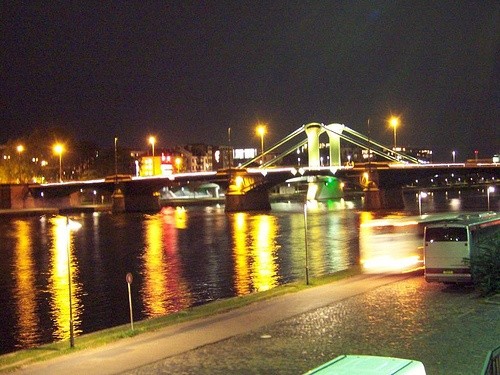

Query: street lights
[53,143,63,181]
[59,211,77,349]
[16,145,23,180]
[388,117,399,160]
[148,136,156,176]
[256,125,264,163]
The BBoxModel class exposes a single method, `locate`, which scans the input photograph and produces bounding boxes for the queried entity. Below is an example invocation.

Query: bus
[423,210,500,285]
